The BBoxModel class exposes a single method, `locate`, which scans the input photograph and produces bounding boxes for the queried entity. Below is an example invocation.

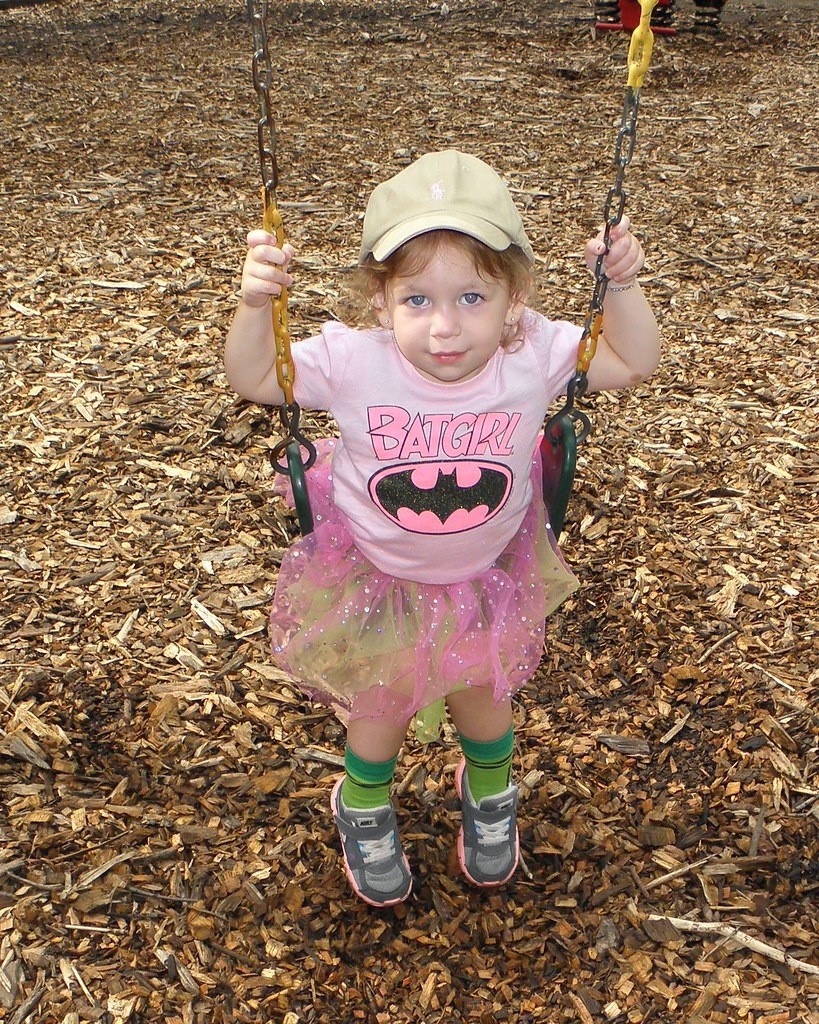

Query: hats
[357,149,536,270]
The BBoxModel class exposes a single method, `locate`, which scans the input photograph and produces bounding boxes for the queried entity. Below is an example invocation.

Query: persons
[222,148,661,908]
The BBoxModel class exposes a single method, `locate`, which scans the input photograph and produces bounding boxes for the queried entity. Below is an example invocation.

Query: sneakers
[454,755,520,887]
[331,773,414,907]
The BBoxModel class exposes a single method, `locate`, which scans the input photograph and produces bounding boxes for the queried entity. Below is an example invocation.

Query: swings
[247,0,657,547]
